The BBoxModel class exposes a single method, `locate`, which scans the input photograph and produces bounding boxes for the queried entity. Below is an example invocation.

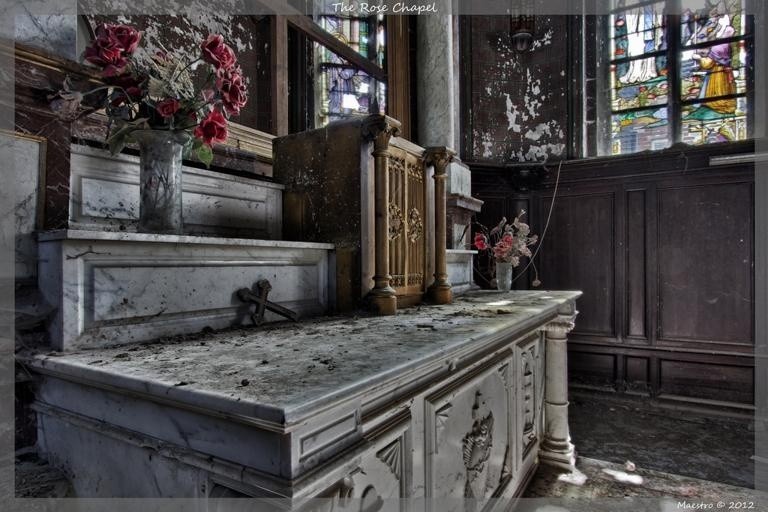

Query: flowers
[64,20,248,206]
[474,209,542,286]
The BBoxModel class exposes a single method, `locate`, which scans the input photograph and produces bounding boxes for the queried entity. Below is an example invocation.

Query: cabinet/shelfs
[272,120,457,320]
[15,229,582,512]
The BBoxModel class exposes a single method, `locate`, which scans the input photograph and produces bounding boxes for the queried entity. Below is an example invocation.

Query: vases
[496,263,513,291]
[139,129,183,234]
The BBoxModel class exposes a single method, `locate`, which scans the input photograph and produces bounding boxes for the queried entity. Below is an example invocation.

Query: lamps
[510,0,536,51]
